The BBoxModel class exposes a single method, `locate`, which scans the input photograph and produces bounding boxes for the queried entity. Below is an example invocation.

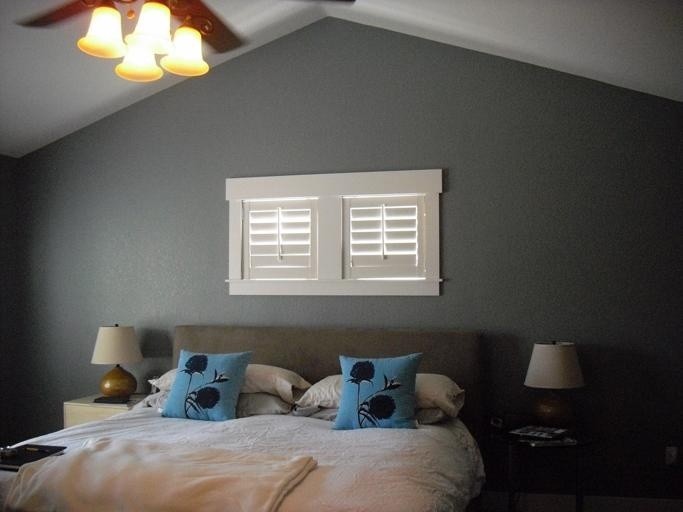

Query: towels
[2,436,317,512]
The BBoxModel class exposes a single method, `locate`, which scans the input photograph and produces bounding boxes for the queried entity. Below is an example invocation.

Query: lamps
[76,0,214,83]
[90,323,144,400]
[521,340,585,423]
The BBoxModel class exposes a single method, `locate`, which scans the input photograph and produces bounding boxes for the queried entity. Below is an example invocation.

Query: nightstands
[509,421,595,496]
[63,394,145,428]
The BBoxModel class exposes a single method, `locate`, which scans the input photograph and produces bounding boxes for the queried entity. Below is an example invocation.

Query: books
[509,425,577,448]
[0,444,67,472]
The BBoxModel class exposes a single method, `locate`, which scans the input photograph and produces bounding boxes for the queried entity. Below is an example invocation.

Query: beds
[0,326,489,512]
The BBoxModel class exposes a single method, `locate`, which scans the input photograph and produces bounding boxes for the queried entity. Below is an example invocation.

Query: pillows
[333,353,423,429]
[161,348,253,422]
[311,408,445,425]
[148,363,312,404]
[139,393,292,417]
[295,374,466,418]
